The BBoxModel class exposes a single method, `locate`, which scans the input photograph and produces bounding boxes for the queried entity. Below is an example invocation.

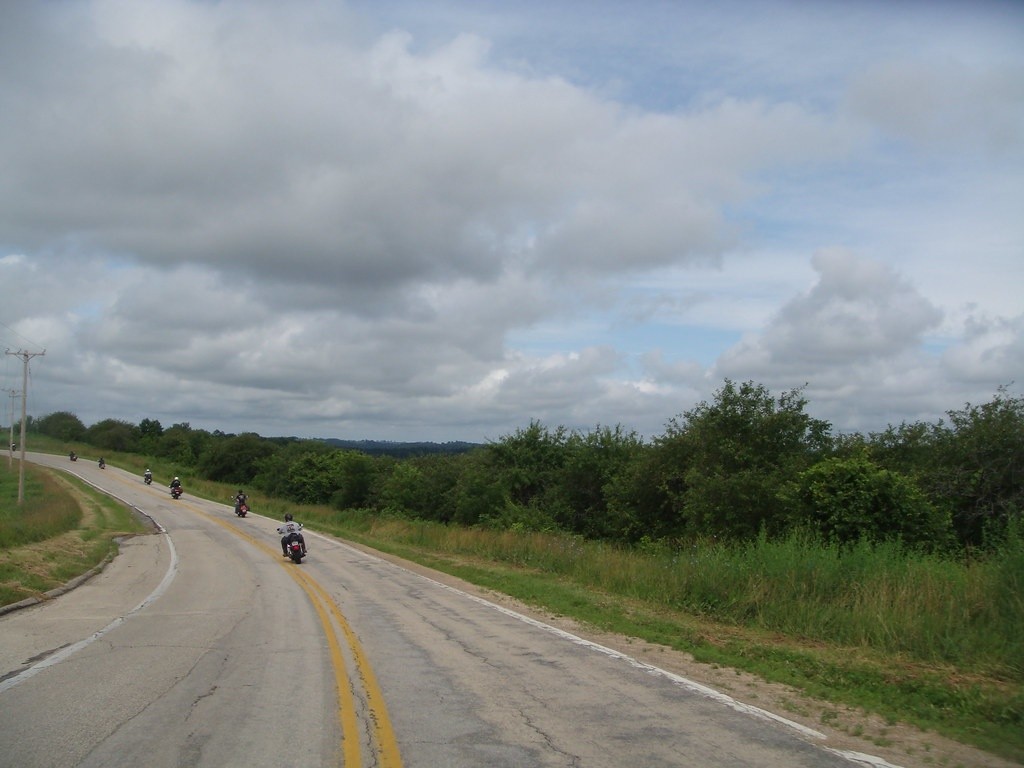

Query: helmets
[147,469,149,472]
[239,490,243,494]
[285,514,292,522]
[175,477,178,480]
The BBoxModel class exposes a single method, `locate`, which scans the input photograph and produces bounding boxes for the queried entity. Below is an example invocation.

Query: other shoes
[303,550,307,553]
[283,553,287,557]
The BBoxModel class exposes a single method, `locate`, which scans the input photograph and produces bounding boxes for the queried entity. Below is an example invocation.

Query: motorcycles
[277,523,306,564]
[231,495,251,518]
[169,484,182,499]
[10,445,16,450]
[70,455,77,461]
[144,474,151,484]
[98,461,105,469]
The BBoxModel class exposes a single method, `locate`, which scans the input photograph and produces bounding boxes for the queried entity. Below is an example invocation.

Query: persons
[144,470,152,483]
[97,457,105,468]
[170,476,181,497]
[12,442,16,448]
[69,451,75,460]
[233,489,248,513]
[279,513,307,557]
